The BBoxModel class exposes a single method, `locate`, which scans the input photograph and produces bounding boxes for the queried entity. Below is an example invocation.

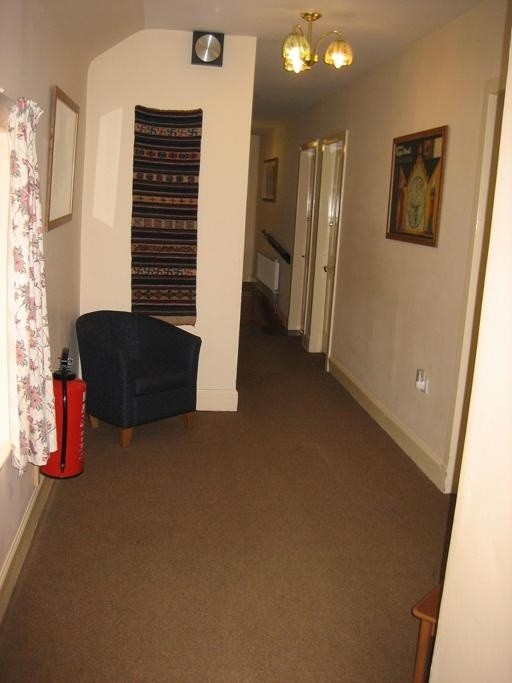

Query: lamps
[282,11,352,74]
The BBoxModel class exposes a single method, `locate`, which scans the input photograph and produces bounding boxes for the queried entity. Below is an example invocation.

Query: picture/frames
[386,125,448,249]
[262,157,278,201]
[45,85,80,234]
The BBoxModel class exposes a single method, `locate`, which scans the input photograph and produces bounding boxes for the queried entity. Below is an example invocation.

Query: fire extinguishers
[39,348,86,479]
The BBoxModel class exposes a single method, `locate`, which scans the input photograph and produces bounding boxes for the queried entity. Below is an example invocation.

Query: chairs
[77,310,202,448]
